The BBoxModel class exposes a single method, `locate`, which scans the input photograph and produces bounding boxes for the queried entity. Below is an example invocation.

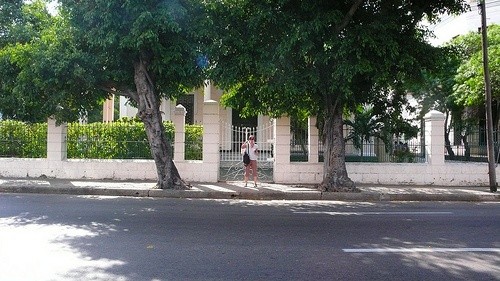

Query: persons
[241,135,259,188]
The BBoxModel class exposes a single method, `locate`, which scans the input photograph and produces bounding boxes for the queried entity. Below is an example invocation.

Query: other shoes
[254,184,259,188]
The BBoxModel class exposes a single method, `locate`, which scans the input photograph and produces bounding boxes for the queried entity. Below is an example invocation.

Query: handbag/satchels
[243,152,250,165]
